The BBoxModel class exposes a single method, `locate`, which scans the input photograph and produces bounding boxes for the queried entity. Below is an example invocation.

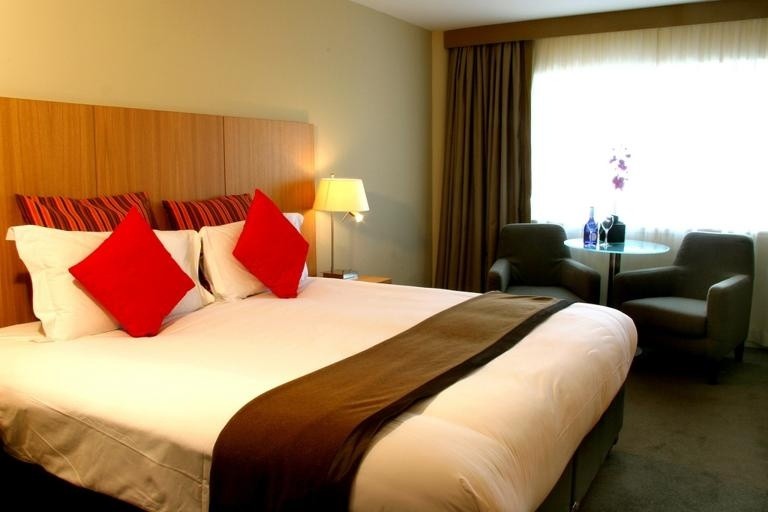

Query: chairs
[487,221,602,304]
[609,231,756,387]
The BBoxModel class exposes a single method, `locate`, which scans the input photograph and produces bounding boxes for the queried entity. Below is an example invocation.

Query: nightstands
[357,276,392,284]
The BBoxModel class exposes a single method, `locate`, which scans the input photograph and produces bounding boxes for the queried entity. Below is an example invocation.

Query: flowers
[607,146,632,191]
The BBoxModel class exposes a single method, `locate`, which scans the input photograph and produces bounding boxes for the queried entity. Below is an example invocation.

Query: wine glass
[584,217,597,248]
[600,216,613,248]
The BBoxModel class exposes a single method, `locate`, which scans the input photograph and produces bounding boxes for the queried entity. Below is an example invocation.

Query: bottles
[582,207,598,246]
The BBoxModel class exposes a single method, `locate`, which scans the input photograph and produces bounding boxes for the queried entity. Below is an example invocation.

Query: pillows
[68,202,197,338]
[161,193,254,230]
[232,188,309,299]
[5,224,218,344]
[13,188,156,233]
[199,212,310,300]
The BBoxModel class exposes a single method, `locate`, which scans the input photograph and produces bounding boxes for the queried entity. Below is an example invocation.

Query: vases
[598,215,625,242]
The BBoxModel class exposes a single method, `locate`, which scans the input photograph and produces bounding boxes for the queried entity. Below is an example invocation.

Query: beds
[0,85,641,512]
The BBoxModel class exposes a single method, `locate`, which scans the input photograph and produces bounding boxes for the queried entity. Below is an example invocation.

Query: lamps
[313,175,370,278]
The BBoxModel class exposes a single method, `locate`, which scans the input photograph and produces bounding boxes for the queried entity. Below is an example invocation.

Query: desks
[561,235,673,306]
